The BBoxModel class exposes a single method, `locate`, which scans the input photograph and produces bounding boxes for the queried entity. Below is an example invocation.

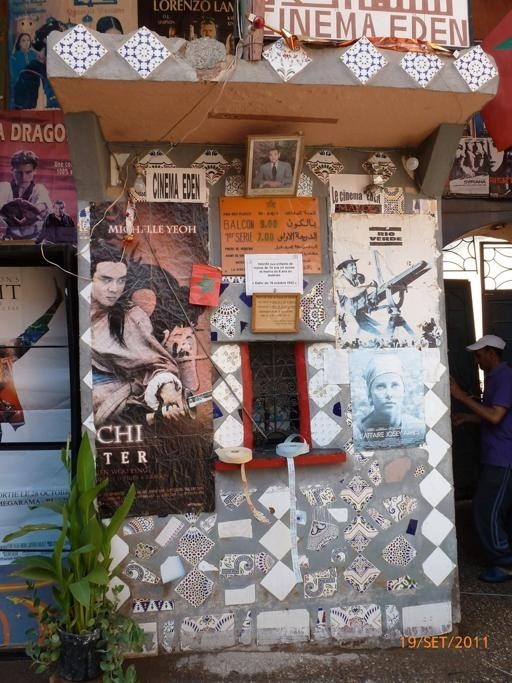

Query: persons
[0,148,54,239]
[476,137,510,192]
[31,198,76,244]
[199,15,218,38]
[354,352,424,444]
[251,143,294,189]
[336,249,381,340]
[9,31,42,109]
[449,330,512,583]
[94,14,123,37]
[87,198,186,429]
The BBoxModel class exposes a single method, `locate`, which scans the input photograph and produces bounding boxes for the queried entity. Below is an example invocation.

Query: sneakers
[478,567,512,583]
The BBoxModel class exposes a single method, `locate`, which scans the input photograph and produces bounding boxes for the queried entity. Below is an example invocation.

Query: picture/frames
[246,133,303,199]
[253,293,300,333]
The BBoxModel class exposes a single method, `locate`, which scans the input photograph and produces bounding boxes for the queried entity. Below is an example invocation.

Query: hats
[365,356,407,393]
[335,254,360,270]
[465,335,507,353]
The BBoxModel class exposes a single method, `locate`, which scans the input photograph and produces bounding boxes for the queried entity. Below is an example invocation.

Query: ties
[272,163,277,179]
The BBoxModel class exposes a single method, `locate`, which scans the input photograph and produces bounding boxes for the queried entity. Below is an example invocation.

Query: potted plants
[2,427,151,683]
